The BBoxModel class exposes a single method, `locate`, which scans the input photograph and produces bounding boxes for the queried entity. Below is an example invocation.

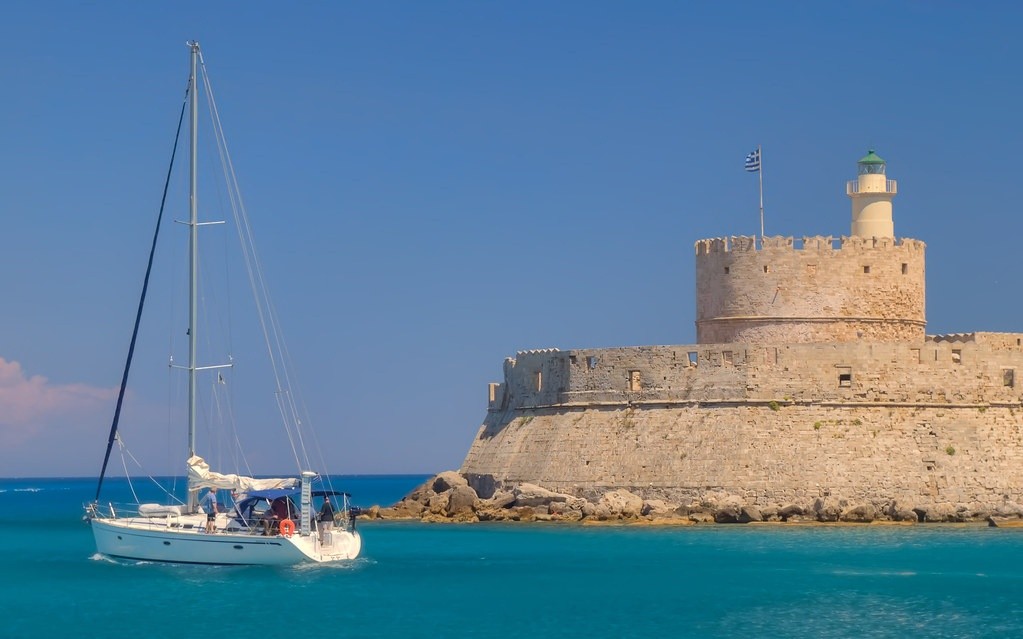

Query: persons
[204,487,217,533]
[316,496,335,545]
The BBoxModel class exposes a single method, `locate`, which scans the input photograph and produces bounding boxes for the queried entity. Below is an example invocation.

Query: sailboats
[81,42,363,566]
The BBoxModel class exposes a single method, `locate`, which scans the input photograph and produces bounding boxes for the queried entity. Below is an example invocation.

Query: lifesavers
[281,519,295,534]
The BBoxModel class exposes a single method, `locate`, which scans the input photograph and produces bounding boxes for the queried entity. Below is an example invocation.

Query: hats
[211,486,216,491]
[324,495,329,499]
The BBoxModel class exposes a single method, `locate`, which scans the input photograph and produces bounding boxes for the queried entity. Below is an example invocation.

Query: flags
[745,149,760,172]
